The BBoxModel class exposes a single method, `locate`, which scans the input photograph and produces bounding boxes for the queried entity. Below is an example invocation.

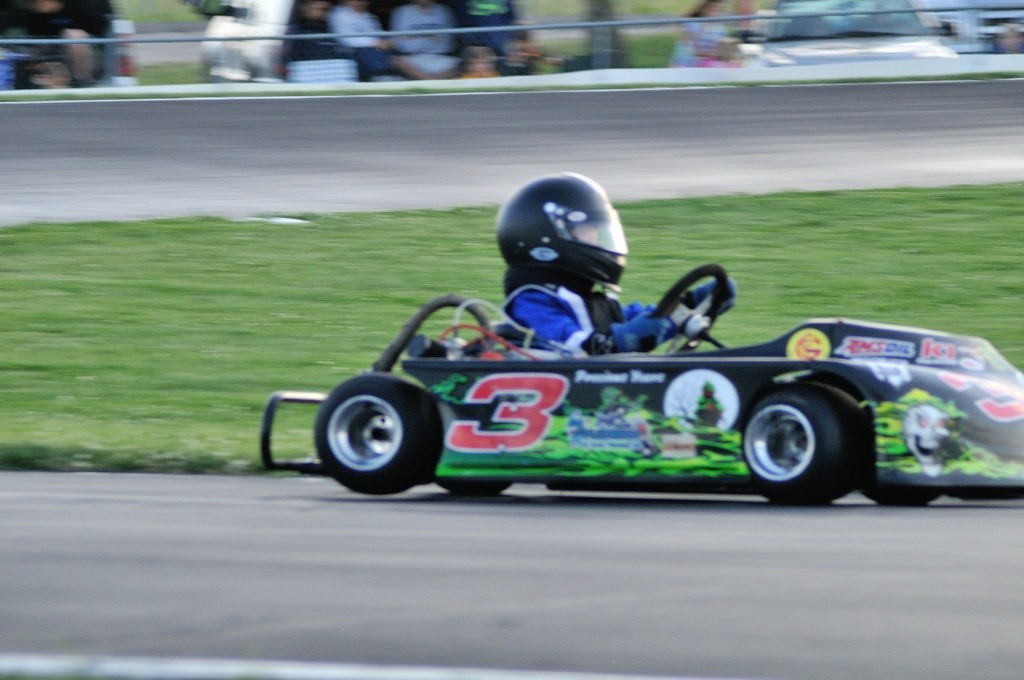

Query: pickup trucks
[1,1,137,89]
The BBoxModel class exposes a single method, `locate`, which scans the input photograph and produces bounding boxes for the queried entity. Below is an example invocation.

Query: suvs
[187,2,514,81]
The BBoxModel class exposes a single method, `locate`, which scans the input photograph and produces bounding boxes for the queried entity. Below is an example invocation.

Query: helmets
[496,171,628,286]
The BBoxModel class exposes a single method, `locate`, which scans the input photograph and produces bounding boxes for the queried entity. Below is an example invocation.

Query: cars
[667,1,959,71]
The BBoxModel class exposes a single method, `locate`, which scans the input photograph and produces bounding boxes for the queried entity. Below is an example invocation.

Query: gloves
[693,276,736,317]
[610,312,672,352]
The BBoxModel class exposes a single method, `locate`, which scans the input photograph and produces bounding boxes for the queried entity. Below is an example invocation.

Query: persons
[0,0,116,93]
[496,171,736,358]
[993,23,1024,54]
[280,0,538,83]
[667,0,779,68]
